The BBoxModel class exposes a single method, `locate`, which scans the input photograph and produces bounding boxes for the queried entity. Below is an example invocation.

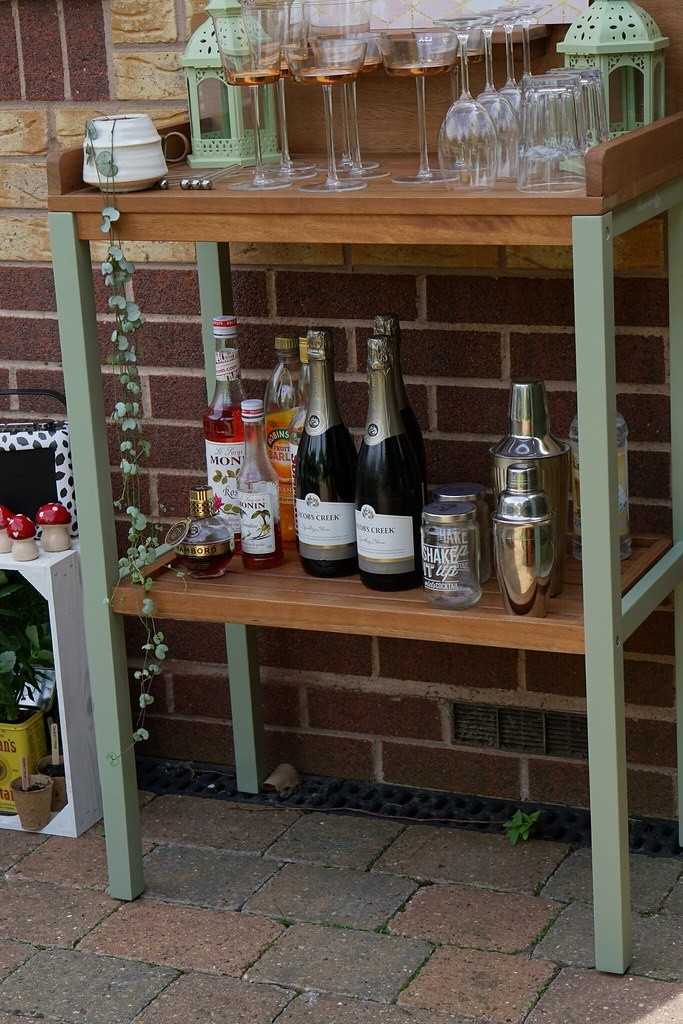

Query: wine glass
[206,1,548,193]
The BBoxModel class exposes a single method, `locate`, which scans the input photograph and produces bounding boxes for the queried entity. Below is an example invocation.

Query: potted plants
[0,569,56,817]
[82,115,169,768]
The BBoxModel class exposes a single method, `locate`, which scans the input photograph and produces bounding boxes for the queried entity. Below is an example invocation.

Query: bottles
[420,502,482,609]
[432,484,489,584]
[296,331,358,579]
[174,486,235,578]
[264,334,304,539]
[236,399,284,571]
[288,337,312,493]
[355,334,419,592]
[569,410,631,563]
[372,317,424,508]
[203,316,248,553]
[491,463,555,618]
[489,379,569,597]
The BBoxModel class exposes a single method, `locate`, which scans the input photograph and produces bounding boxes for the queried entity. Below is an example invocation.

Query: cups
[516,67,611,193]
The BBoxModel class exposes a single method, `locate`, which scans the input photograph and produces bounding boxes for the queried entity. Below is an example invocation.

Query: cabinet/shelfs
[0,538,103,838]
[44,113,682,977]
